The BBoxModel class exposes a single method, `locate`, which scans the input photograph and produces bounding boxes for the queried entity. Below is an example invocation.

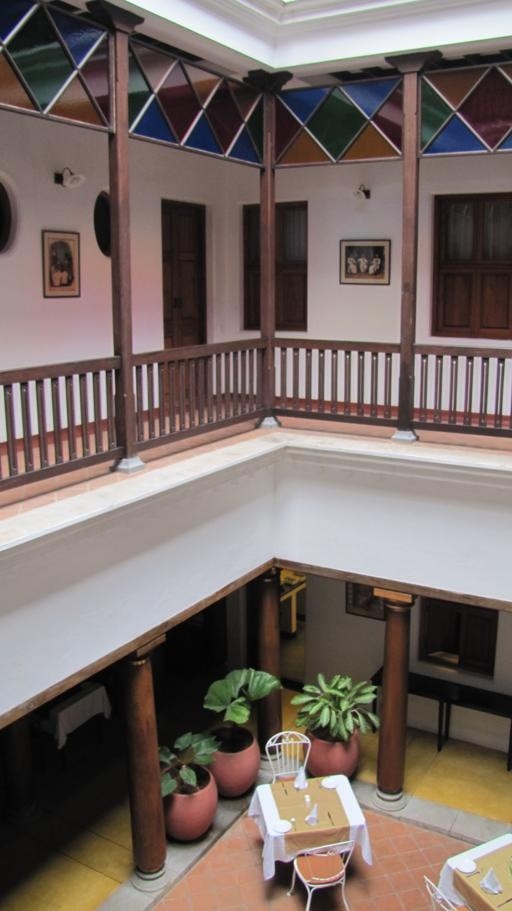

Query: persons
[345,253,358,275]
[367,254,381,276]
[356,253,370,274]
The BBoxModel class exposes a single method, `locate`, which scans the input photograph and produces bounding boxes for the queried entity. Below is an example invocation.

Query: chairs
[286,840,356,911]
[264,730,312,785]
[423,874,470,911]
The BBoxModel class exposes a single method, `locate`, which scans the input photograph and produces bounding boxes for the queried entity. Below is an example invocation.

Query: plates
[272,819,293,834]
[454,856,477,874]
[321,776,341,789]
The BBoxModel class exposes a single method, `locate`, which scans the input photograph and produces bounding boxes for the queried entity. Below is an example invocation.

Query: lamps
[351,183,371,203]
[54,166,86,191]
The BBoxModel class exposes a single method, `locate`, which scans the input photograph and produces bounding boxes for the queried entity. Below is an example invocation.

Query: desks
[370,665,512,771]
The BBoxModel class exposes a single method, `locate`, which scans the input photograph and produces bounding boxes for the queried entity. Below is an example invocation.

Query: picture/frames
[345,580,386,621]
[339,239,391,285]
[41,230,81,298]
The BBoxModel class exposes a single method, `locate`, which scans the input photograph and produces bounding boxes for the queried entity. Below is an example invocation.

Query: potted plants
[290,671,382,779]
[157,667,285,842]
